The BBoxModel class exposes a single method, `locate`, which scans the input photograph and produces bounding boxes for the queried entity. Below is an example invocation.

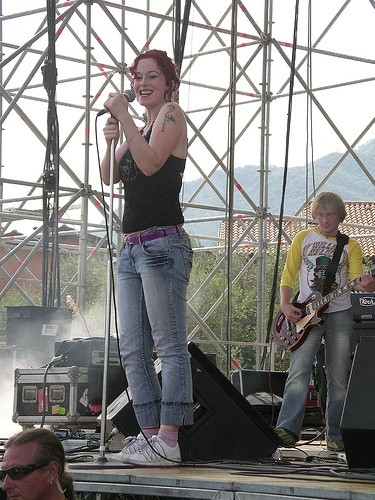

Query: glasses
[0,459,50,483]
[314,211,337,220]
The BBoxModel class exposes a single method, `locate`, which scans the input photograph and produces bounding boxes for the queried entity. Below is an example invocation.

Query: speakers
[339,341,375,473]
[97,341,284,463]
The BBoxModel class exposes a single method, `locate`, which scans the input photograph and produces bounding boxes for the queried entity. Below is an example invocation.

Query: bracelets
[128,127,138,143]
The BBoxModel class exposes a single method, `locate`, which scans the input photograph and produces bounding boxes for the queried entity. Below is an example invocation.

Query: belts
[124,225,183,246]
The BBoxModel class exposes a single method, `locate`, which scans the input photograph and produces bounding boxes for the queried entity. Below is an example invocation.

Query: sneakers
[109,433,148,462]
[122,435,183,467]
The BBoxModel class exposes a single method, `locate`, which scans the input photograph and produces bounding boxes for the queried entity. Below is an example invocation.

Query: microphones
[97,90,136,116]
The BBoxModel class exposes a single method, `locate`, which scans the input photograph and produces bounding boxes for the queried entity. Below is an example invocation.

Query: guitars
[273,267,375,353]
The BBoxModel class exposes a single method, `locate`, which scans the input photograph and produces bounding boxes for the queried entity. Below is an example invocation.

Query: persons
[101,49,193,466]
[0,428,76,500]
[272,192,375,453]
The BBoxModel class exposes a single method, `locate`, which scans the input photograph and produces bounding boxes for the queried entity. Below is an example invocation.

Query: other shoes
[326,440,345,451]
[274,427,299,448]
